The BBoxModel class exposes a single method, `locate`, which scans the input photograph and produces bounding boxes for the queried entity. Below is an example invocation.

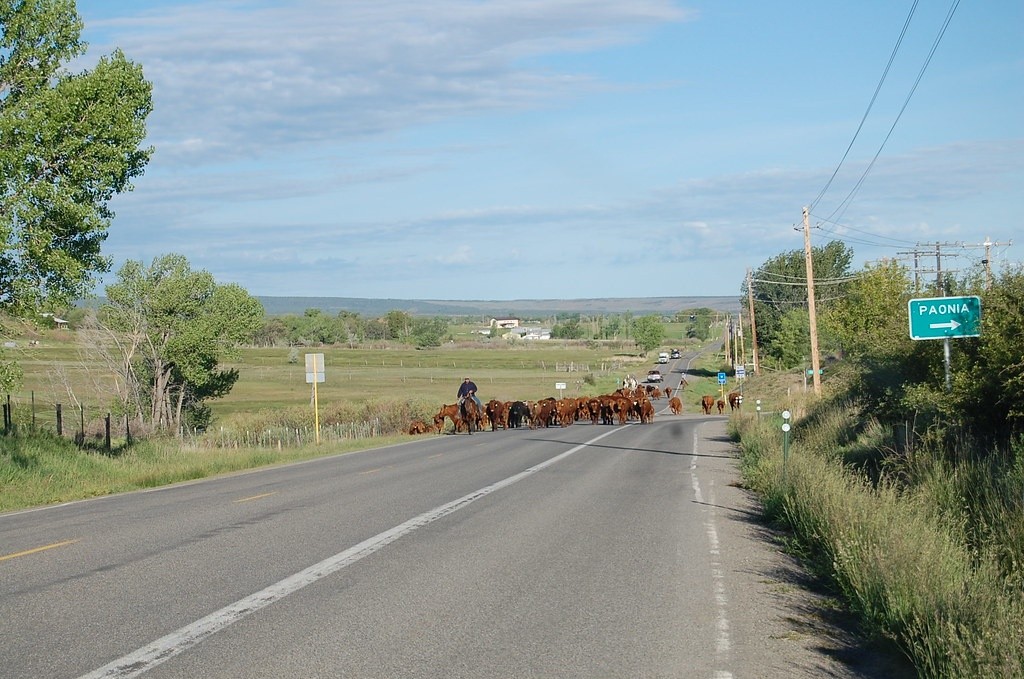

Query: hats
[465,377,470,381]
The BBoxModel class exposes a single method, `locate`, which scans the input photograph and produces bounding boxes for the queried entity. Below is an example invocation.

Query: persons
[455,376,485,420]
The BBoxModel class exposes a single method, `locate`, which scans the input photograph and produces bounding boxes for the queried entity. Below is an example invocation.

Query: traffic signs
[907,295,983,340]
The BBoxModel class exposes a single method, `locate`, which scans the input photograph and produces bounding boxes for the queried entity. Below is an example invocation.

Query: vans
[658,352,669,363]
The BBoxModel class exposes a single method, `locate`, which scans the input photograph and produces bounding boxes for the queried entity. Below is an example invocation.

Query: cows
[408,384,673,436]
[728,392,744,412]
[680,378,689,390]
[667,396,683,417]
[716,400,726,415]
[701,395,715,415]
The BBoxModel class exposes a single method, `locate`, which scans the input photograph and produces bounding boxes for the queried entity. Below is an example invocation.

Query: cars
[647,371,662,384]
[670,351,681,358]
